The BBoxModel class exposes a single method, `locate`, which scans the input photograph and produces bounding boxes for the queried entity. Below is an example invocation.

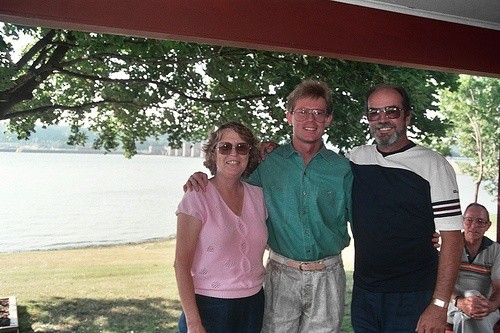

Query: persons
[173,120,269,333]
[446,202,500,333]
[256,85,465,333]
[183,78,441,333]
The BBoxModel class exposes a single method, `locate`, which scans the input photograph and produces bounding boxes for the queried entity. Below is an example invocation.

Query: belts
[269,252,341,271]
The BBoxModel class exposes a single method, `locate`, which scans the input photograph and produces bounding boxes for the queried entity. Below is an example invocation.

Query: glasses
[215,142,252,155]
[291,108,330,123]
[366,106,408,121]
[463,216,489,228]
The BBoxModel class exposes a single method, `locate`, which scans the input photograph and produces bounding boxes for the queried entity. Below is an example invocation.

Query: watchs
[452,296,461,307]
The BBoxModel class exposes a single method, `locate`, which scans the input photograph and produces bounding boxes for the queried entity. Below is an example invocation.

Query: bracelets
[430,297,449,309]
